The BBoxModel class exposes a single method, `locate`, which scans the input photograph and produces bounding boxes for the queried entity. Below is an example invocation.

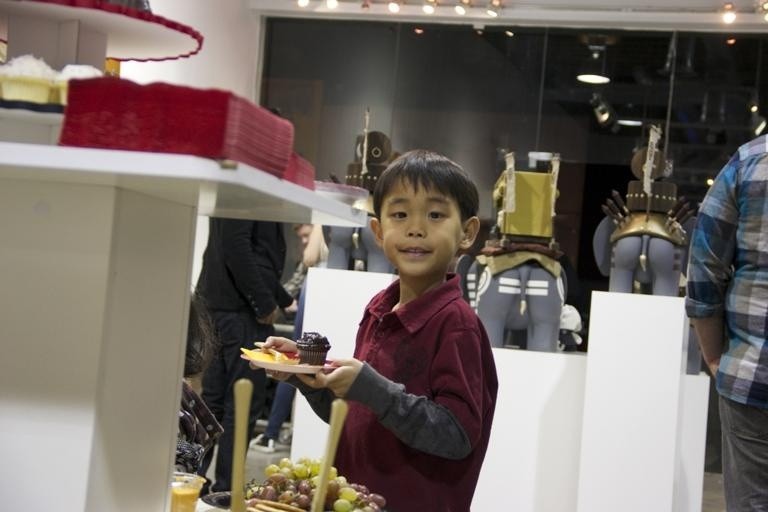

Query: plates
[201,490,233,509]
[240,347,342,374]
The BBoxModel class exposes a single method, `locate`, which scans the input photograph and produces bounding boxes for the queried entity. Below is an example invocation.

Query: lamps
[749,114,765,137]
[720,0,767,25]
[746,39,763,114]
[590,87,611,124]
[291,0,505,24]
[575,31,615,85]
[613,100,644,129]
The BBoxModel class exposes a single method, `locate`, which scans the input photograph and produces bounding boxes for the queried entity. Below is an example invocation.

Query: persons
[249,223,331,454]
[685,134,768,512]
[193,216,300,511]
[247,150,500,512]
[282,223,314,299]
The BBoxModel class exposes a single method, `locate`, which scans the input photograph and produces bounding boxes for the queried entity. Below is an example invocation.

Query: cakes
[0,54,58,105]
[59,63,103,106]
[295,331,331,367]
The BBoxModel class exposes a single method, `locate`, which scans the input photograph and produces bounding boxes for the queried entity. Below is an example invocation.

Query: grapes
[245,457,386,512]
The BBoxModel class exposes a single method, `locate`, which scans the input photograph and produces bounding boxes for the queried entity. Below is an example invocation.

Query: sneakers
[250,433,275,454]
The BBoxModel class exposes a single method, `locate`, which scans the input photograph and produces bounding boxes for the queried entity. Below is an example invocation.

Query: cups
[170,470,207,512]
